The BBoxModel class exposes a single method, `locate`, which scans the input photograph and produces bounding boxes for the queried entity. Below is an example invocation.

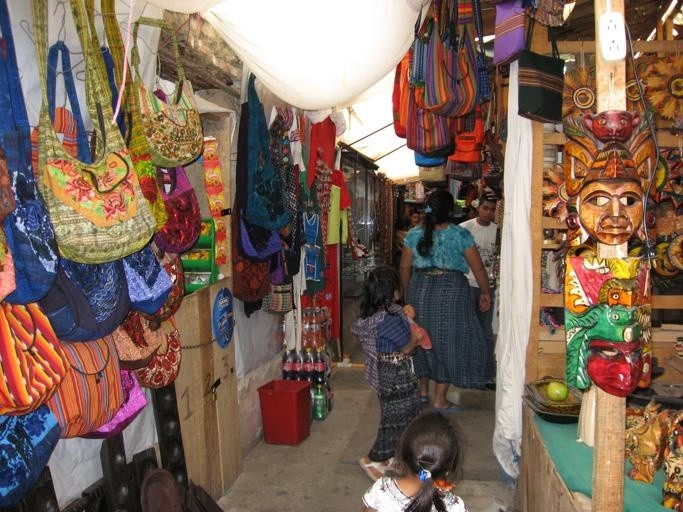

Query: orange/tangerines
[547,381,568,402]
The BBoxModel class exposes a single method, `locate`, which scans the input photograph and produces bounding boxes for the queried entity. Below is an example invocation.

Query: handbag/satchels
[492,0,525,64]
[1,0,204,508]
[262,282,292,313]
[516,49,563,122]
[393,19,489,184]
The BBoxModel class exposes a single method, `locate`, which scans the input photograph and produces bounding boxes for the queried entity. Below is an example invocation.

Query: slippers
[433,404,462,414]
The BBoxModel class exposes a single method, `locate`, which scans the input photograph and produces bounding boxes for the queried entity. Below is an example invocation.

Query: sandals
[358,458,405,483]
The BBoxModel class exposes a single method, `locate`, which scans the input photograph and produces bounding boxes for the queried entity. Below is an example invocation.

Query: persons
[408,211,421,228]
[456,192,501,390]
[356,265,424,483]
[359,410,467,512]
[396,190,490,413]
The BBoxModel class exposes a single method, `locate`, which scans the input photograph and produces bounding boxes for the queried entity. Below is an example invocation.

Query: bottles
[300,307,331,347]
[308,384,332,421]
[283,346,331,386]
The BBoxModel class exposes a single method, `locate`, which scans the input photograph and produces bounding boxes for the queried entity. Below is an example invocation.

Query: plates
[522,379,582,424]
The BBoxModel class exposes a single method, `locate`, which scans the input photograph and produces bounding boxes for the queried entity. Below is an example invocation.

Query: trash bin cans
[256,381,312,446]
